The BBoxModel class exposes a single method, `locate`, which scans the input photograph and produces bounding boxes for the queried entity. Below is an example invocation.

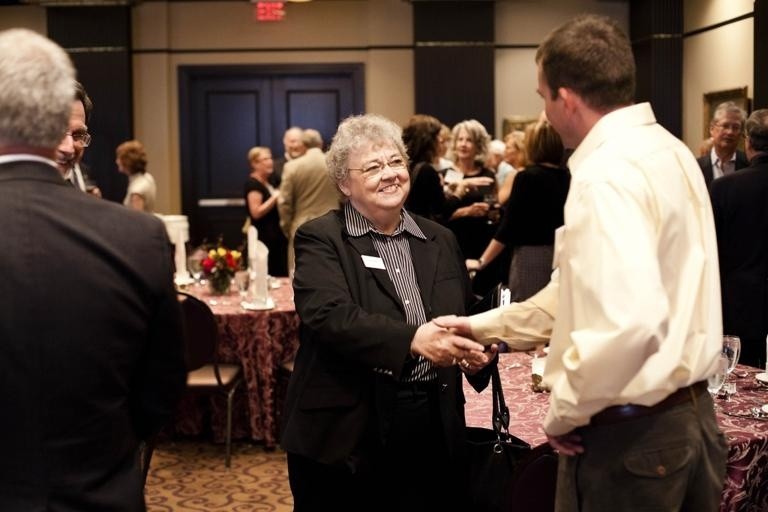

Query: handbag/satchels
[444,425,532,512]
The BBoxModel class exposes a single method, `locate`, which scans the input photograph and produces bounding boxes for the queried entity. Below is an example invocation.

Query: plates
[760,403,768,414]
[755,372,768,383]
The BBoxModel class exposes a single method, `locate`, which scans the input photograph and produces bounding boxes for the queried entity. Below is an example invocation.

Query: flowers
[201,249,244,292]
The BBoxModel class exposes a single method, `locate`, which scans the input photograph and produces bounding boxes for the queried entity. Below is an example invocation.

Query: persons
[432,16,729,511]
[0,27,183,512]
[284,114,496,510]
[70,162,101,197]
[51,80,93,175]
[693,100,745,183]
[710,108,768,371]
[269,126,304,189]
[242,145,289,277]
[115,139,157,216]
[275,129,342,275]
[404,106,567,303]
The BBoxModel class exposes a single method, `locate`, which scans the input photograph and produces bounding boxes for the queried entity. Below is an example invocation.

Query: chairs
[173,291,245,469]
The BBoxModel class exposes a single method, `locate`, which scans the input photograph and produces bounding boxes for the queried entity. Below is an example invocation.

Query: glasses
[714,123,742,130]
[348,158,410,179]
[64,130,92,149]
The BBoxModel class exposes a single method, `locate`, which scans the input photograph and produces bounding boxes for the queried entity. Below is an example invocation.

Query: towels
[244,224,270,304]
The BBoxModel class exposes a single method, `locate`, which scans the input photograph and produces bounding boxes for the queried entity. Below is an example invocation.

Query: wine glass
[707,353,728,412]
[235,272,251,311]
[484,193,498,226]
[721,336,741,408]
[188,257,204,299]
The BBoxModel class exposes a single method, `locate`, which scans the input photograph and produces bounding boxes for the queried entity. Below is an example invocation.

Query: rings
[452,357,456,365]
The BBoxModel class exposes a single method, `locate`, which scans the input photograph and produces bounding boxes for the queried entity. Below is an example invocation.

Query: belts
[598,379,708,416]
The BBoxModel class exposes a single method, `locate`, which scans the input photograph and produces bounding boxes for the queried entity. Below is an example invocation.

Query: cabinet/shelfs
[197,204,247,248]
